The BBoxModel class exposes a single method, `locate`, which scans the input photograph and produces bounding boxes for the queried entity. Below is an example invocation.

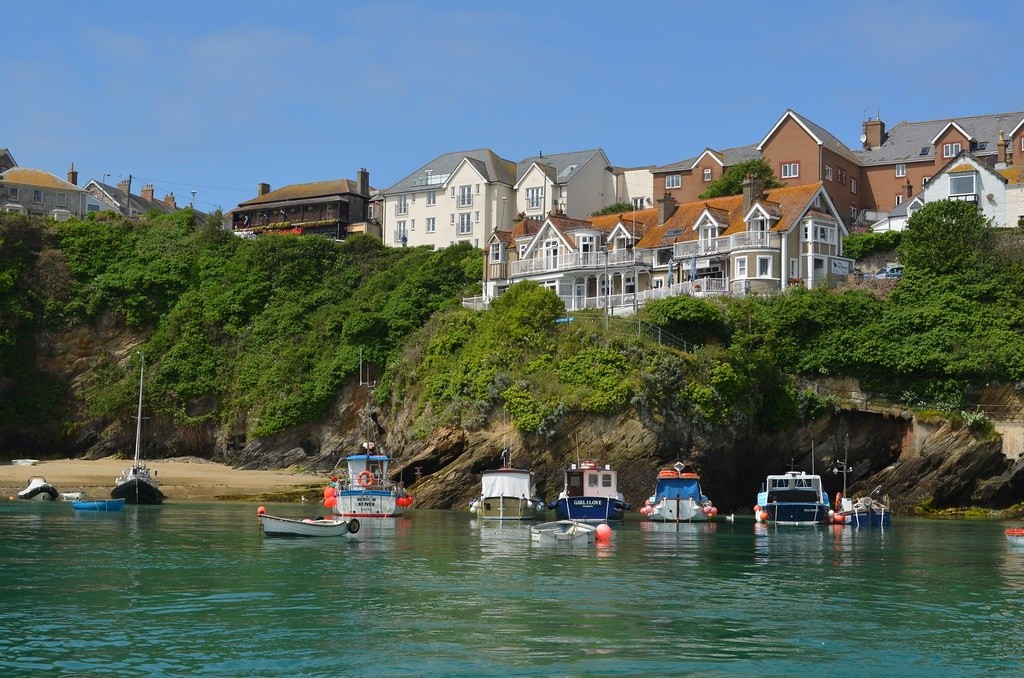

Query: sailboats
[332,348,407,517]
[111,350,170,505]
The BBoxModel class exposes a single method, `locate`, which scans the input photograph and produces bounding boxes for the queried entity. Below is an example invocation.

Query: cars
[875,267,904,277]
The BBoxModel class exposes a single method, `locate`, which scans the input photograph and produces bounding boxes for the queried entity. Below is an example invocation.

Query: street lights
[482,250,489,296]
[601,245,609,331]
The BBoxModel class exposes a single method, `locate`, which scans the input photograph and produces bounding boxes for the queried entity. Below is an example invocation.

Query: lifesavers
[357,471,375,486]
[835,492,841,510]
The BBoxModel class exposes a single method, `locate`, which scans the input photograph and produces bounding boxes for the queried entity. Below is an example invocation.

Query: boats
[836,496,893,526]
[647,464,711,520]
[11,459,39,466]
[554,459,626,522]
[62,491,86,501]
[256,506,360,537]
[476,468,541,519]
[756,471,830,526]
[1004,529,1024,547]
[18,476,59,501]
[530,520,597,544]
[72,498,126,510]
